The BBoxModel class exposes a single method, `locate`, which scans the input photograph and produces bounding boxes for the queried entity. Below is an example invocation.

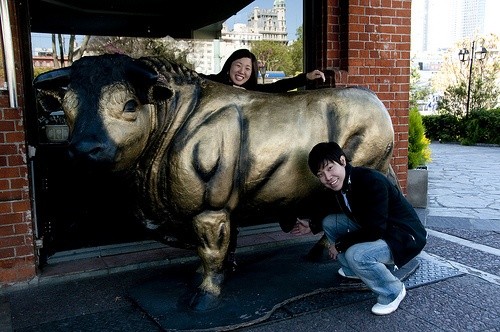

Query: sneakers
[372,283,406,315]
[338,268,361,279]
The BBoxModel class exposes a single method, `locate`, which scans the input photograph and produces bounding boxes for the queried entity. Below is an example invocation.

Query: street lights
[459,39,487,121]
[260,63,267,85]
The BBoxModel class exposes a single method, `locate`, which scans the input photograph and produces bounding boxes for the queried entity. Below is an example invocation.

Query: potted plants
[407,104,433,209]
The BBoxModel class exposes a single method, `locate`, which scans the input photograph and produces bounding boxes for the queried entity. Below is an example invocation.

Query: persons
[198,49,325,272]
[291,142,427,316]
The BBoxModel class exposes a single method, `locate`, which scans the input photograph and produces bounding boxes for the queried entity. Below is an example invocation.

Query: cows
[34,53,401,316]
